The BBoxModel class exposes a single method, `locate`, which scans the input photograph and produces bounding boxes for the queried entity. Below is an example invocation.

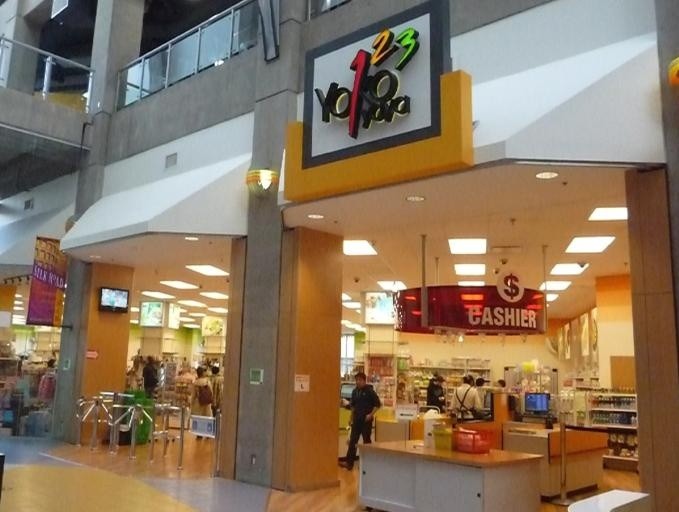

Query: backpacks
[198,385,213,407]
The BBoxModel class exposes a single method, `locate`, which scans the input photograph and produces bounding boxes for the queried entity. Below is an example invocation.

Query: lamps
[667,56,679,89]
[246,169,279,199]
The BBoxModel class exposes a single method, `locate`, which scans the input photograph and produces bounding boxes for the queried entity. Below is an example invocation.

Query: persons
[143,355,219,441]
[427,372,505,426]
[339,372,380,470]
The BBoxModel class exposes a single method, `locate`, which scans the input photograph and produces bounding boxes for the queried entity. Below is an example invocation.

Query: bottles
[559,411,585,427]
[592,397,636,426]
[546,413,553,429]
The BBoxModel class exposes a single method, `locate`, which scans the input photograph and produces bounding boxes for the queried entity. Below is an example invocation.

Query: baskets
[453,424,493,455]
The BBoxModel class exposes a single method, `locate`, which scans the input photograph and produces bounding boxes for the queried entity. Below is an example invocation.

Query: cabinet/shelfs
[128,352,225,416]
[339,353,639,512]
[0,349,59,428]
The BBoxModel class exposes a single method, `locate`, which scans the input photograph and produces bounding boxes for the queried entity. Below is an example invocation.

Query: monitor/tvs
[525,392,550,415]
[98,286,129,313]
[340,383,358,401]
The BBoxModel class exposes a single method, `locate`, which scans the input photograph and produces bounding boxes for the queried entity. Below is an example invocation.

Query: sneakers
[338,462,353,471]
